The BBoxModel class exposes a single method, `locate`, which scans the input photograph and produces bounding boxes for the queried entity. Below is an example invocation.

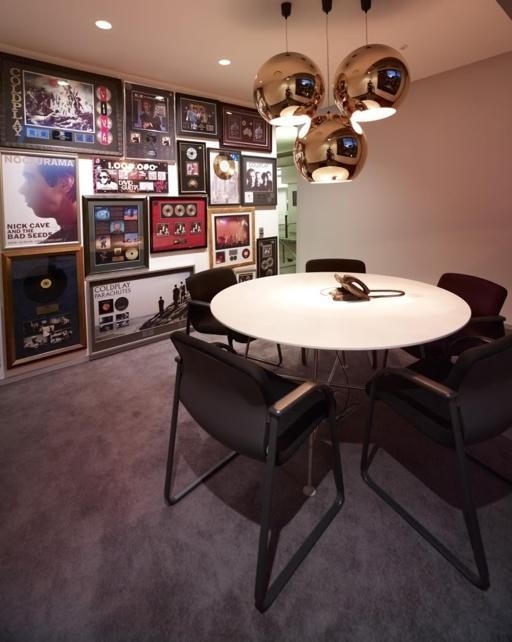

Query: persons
[296,159,312,181]
[173,284,180,308]
[26,85,83,127]
[18,156,78,243]
[96,170,118,190]
[179,281,185,303]
[254,87,274,120]
[367,79,373,94]
[140,98,162,130]
[230,122,240,136]
[339,80,348,113]
[326,146,333,160]
[23,317,72,347]
[255,125,263,140]
[184,103,215,131]
[246,168,273,191]
[285,86,292,100]
[158,296,164,316]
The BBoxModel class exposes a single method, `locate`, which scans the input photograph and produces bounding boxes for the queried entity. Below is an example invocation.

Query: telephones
[334,274,372,300]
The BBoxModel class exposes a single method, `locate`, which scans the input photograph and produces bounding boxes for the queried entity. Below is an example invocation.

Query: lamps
[253,2,325,127]
[333,0,410,123]
[293,0,368,184]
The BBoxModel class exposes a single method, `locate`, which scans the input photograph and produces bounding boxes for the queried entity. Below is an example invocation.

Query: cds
[125,247,139,260]
[187,148,197,160]
[186,204,196,216]
[162,205,173,217]
[174,205,185,216]
